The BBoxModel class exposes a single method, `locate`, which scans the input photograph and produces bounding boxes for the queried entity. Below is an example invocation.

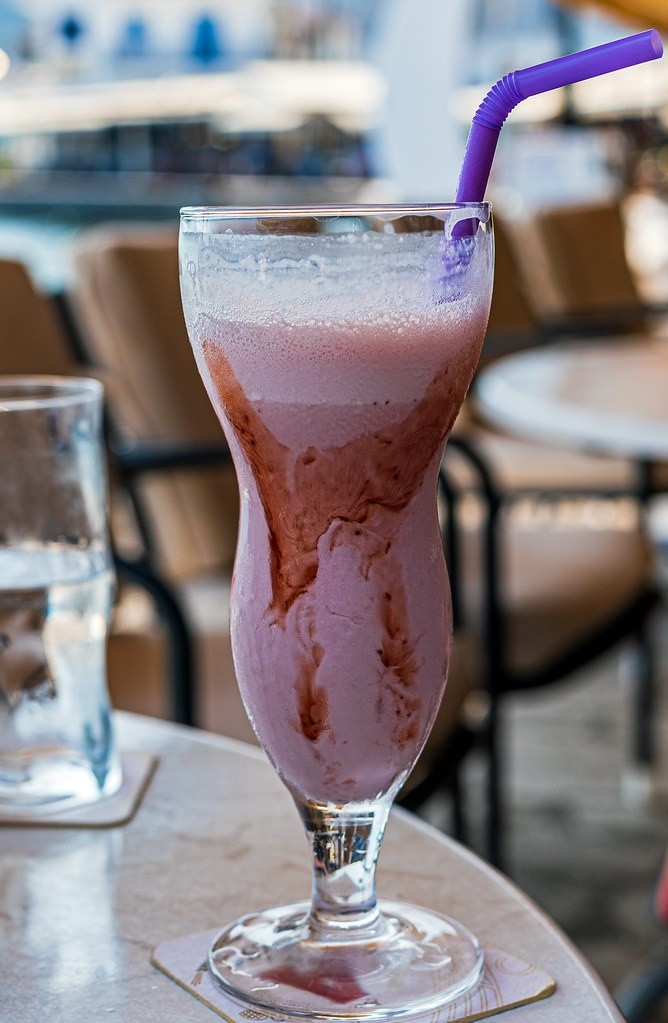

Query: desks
[480,333,668,462]
[0,708,629,1023]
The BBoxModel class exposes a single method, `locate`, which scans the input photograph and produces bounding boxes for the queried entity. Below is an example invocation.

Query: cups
[1,373,125,820]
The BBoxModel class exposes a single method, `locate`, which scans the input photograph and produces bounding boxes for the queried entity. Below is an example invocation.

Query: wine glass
[177,197,494,1022]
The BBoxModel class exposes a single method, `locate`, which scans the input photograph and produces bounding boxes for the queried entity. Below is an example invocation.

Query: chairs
[0,200,668,873]
